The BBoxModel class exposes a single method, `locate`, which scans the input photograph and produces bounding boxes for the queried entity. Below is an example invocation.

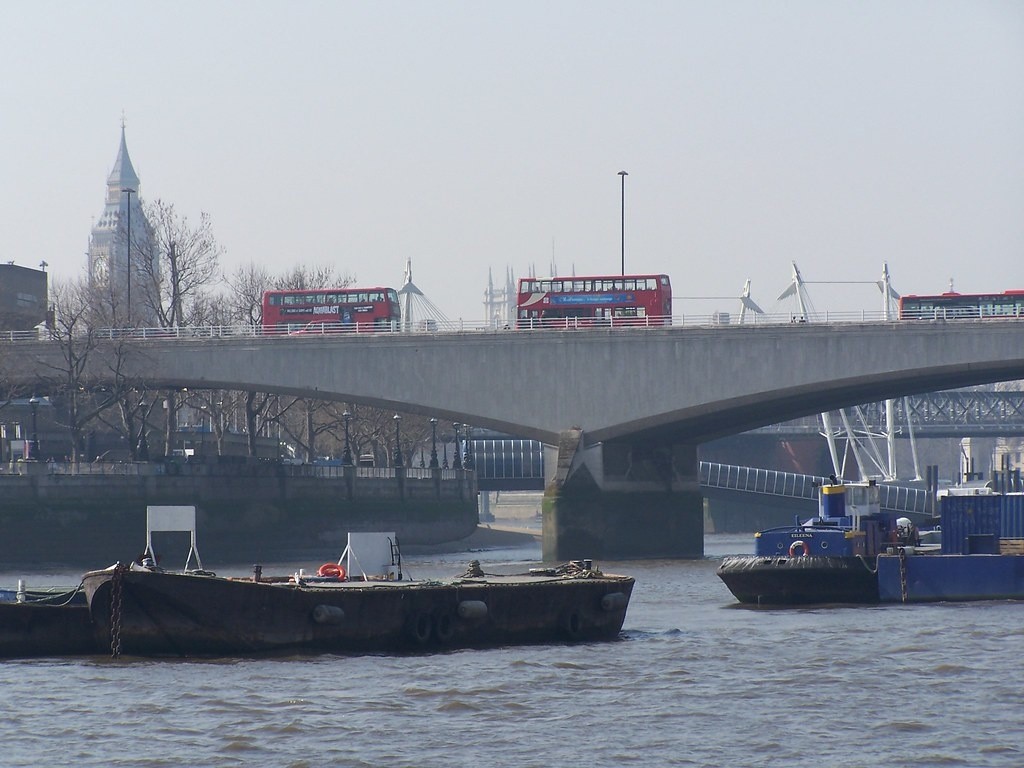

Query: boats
[715,466,944,603]
[875,454,1024,603]
[82,560,635,656]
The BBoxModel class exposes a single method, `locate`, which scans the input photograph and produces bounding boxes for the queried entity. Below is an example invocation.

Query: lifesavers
[788,539,811,560]
[317,562,346,583]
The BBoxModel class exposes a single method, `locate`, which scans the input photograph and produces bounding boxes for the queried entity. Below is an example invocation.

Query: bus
[897,289,1024,320]
[516,273,673,329]
[262,286,402,332]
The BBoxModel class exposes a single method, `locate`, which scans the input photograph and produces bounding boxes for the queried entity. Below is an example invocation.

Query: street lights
[134,399,149,461]
[121,186,135,328]
[24,394,41,460]
[342,410,354,464]
[452,421,476,473]
[616,170,628,314]
[393,412,403,469]
[429,417,441,469]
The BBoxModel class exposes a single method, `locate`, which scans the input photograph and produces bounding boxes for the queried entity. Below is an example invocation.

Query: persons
[792,316,806,323]
[16,456,23,476]
[64,455,71,473]
[535,287,540,292]
